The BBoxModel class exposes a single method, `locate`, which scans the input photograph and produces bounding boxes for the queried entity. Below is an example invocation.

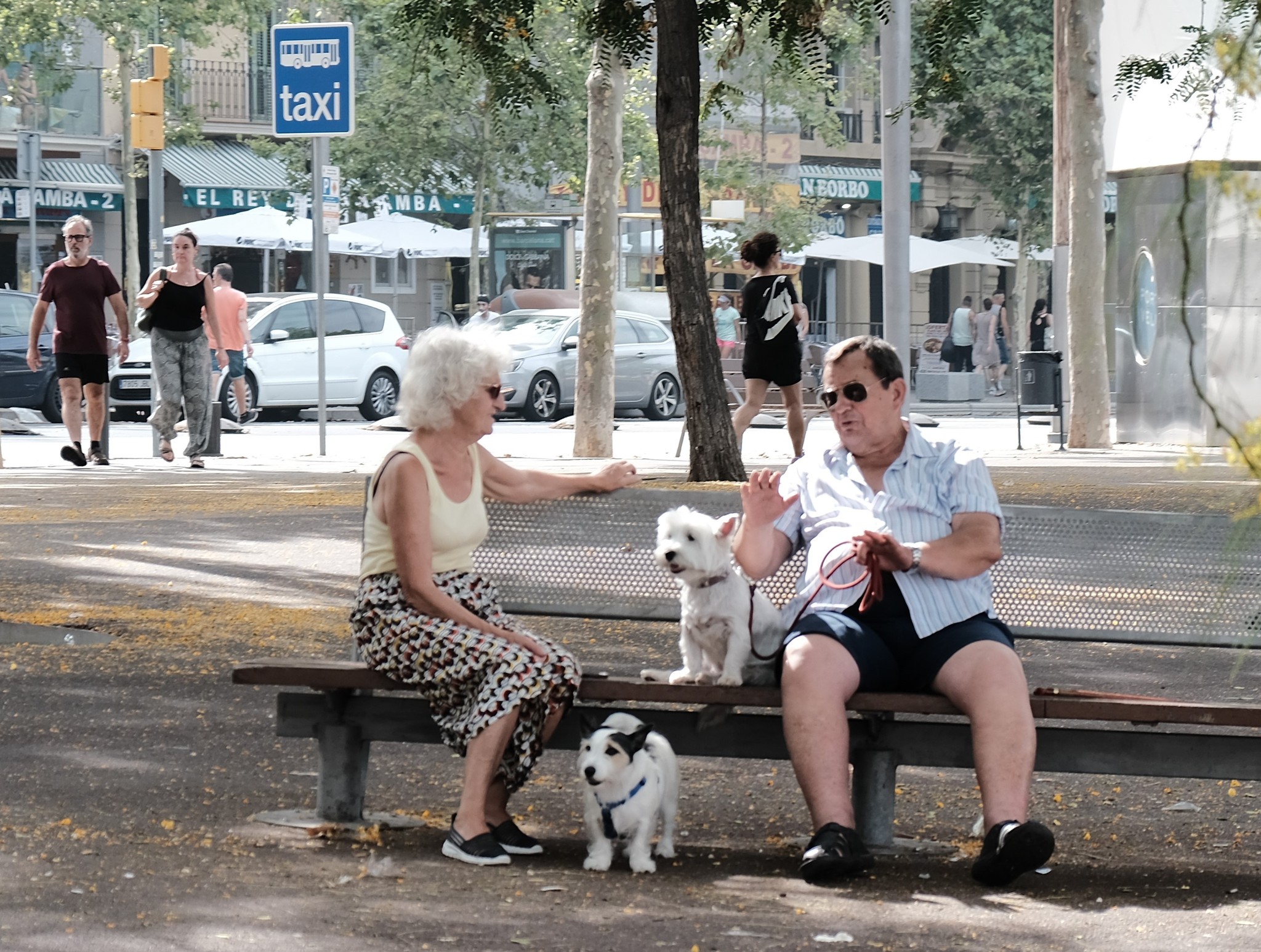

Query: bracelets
[216,346,225,349]
[119,338,129,344]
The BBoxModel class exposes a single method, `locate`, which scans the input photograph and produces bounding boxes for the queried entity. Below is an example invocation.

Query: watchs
[901,543,921,576]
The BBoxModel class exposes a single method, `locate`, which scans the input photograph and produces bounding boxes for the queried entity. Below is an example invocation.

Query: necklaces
[176,266,192,285]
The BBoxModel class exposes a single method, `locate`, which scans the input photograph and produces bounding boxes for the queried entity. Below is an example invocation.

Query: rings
[123,355,127,357]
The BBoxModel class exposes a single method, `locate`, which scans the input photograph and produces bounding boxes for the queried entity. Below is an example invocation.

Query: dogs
[575,711,678,874]
[638,501,786,685]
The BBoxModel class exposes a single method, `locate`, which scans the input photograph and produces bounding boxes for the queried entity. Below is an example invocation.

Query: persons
[712,293,741,360]
[135,227,230,469]
[729,232,808,463]
[463,294,500,325]
[201,263,259,427]
[25,214,131,466]
[499,273,521,295]
[946,288,1053,396]
[520,265,541,289]
[346,326,642,866]
[732,334,1057,892]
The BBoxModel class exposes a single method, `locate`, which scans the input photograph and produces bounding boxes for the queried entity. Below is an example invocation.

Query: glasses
[63,234,85,242]
[479,383,502,401]
[818,377,886,409]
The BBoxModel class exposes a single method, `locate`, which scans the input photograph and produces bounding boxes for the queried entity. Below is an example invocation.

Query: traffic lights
[44,106,69,132]
[145,43,169,81]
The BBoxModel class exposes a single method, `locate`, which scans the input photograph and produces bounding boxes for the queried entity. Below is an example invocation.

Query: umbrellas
[161,204,1053,334]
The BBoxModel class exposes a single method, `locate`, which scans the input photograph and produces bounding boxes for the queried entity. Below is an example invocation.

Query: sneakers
[237,411,259,427]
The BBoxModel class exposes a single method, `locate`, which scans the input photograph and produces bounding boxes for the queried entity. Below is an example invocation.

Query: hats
[717,295,731,304]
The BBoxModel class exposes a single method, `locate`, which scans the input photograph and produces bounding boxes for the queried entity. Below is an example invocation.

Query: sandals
[797,820,877,878]
[158,437,175,462]
[190,456,204,468]
[969,816,1054,887]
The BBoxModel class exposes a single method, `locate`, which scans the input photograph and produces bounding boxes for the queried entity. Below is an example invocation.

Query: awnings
[0,157,125,219]
[330,147,495,214]
[137,139,315,211]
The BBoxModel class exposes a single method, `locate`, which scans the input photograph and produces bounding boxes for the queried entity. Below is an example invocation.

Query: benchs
[676,358,829,457]
[232,479,1261,855]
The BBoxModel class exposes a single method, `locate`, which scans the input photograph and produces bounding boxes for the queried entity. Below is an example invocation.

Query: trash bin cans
[1017,351,1063,413]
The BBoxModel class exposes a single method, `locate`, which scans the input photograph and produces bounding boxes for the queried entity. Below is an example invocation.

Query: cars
[434,309,684,424]
[0,288,86,424]
[106,292,408,426]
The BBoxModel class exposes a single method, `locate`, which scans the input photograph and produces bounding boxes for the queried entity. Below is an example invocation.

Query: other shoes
[60,444,87,466]
[450,813,543,854]
[441,826,511,866]
[88,440,110,465]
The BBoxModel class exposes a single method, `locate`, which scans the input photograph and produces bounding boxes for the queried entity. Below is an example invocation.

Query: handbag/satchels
[136,304,154,332]
[939,336,957,363]
[757,276,797,344]
